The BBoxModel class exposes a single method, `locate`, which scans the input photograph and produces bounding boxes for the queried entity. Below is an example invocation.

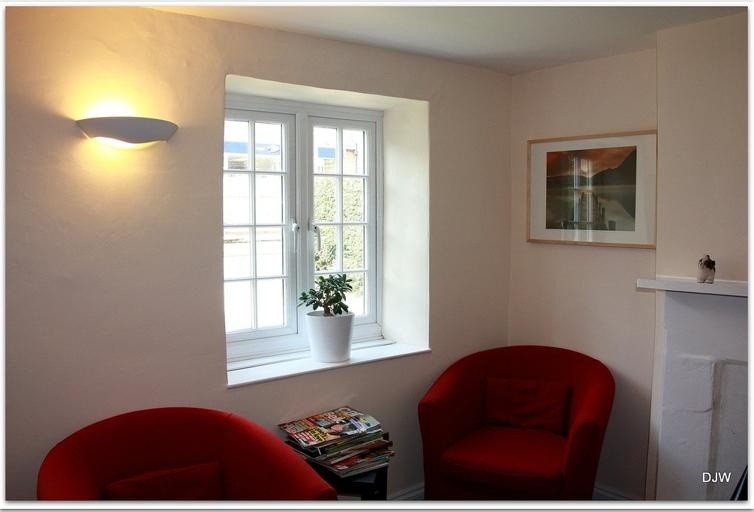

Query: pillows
[99,460,225,499]
[483,373,574,432]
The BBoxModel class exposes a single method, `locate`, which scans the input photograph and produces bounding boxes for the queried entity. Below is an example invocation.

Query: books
[277,406,396,479]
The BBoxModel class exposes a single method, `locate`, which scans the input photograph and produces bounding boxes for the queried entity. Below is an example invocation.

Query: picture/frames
[526,128,656,249]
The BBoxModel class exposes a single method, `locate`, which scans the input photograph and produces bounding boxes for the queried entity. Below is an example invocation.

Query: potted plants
[300,273,357,362]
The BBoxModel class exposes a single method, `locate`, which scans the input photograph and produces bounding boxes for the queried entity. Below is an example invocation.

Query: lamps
[73,116,179,151]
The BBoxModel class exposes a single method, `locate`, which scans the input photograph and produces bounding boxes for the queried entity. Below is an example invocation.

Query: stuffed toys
[697,254,716,283]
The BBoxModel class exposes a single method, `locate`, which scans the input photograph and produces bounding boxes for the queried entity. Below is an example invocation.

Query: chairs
[415,345,617,500]
[36,406,336,498]
